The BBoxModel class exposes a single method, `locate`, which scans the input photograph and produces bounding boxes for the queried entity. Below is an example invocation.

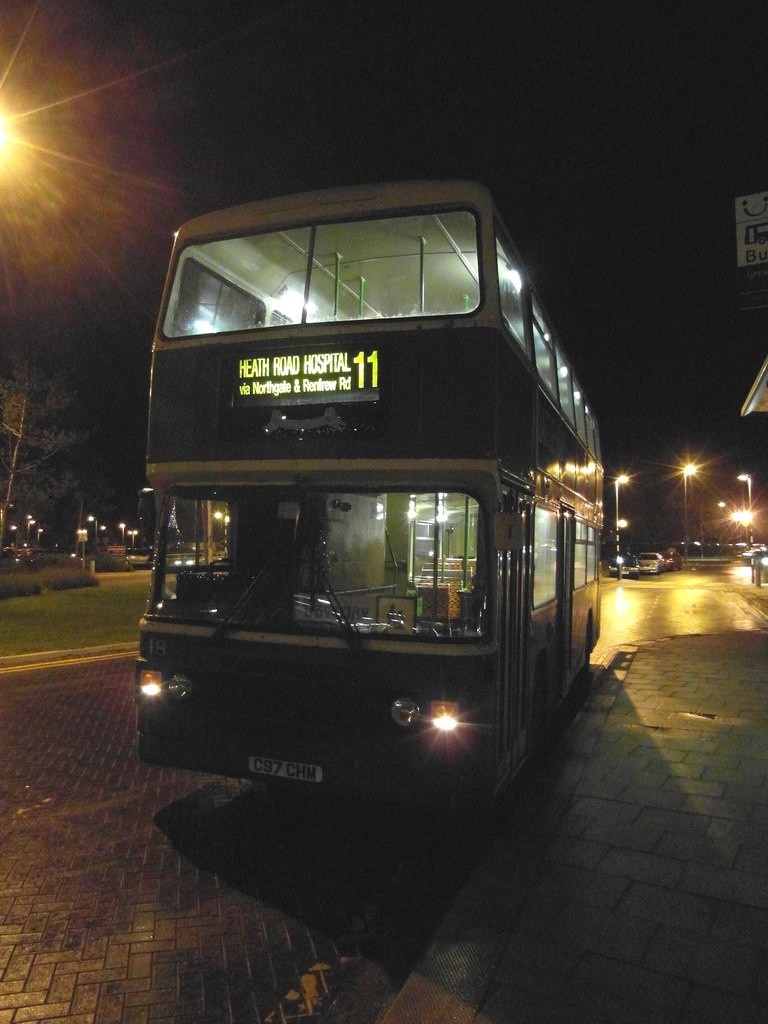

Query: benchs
[125,555,152,570]
[416,553,475,617]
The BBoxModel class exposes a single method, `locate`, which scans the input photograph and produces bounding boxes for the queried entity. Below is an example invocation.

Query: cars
[659,552,681,572]
[609,553,640,579]
[637,553,665,574]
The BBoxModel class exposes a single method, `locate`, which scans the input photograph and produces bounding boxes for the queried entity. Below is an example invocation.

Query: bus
[135,176,606,817]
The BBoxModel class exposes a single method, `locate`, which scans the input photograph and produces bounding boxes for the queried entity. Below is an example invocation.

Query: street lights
[132,531,138,548]
[28,520,35,532]
[89,517,97,542]
[615,477,629,551]
[738,474,754,582]
[37,529,43,541]
[120,524,125,542]
[684,466,695,564]
